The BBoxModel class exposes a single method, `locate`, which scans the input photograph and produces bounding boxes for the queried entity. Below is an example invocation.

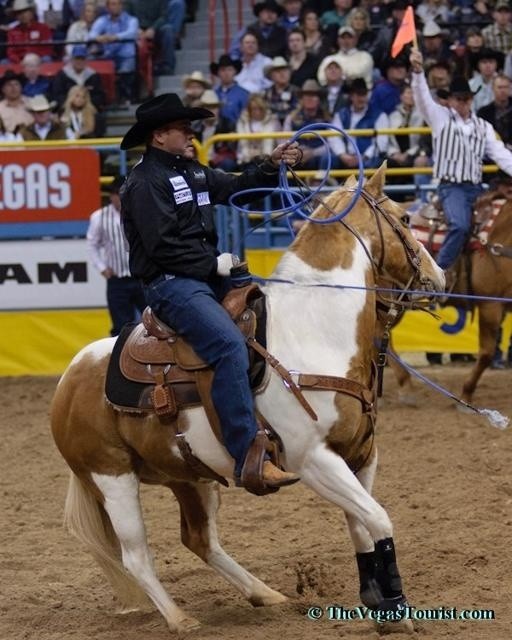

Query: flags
[390,6,417,58]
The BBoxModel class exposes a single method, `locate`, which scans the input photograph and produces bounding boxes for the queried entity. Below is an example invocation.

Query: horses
[374,193,512,416]
[46,156,450,636]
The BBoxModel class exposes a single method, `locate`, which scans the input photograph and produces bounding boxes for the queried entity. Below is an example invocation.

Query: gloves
[216,252,240,277]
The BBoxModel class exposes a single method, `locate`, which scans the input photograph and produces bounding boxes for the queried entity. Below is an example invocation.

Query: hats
[101,175,126,193]
[72,47,87,59]
[5,0,35,16]
[189,90,227,108]
[0,69,27,87]
[182,1,510,102]
[24,94,59,112]
[120,93,215,150]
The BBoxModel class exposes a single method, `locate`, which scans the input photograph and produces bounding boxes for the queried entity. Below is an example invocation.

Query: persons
[490,323,512,369]
[118,92,304,491]
[408,47,512,277]
[181,0,511,209]
[88,169,154,341]
[1,0,198,142]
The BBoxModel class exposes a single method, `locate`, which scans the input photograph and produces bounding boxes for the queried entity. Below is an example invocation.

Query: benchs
[0,0,180,109]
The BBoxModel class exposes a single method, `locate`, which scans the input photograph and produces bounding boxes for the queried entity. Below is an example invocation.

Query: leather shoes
[233,460,301,487]
